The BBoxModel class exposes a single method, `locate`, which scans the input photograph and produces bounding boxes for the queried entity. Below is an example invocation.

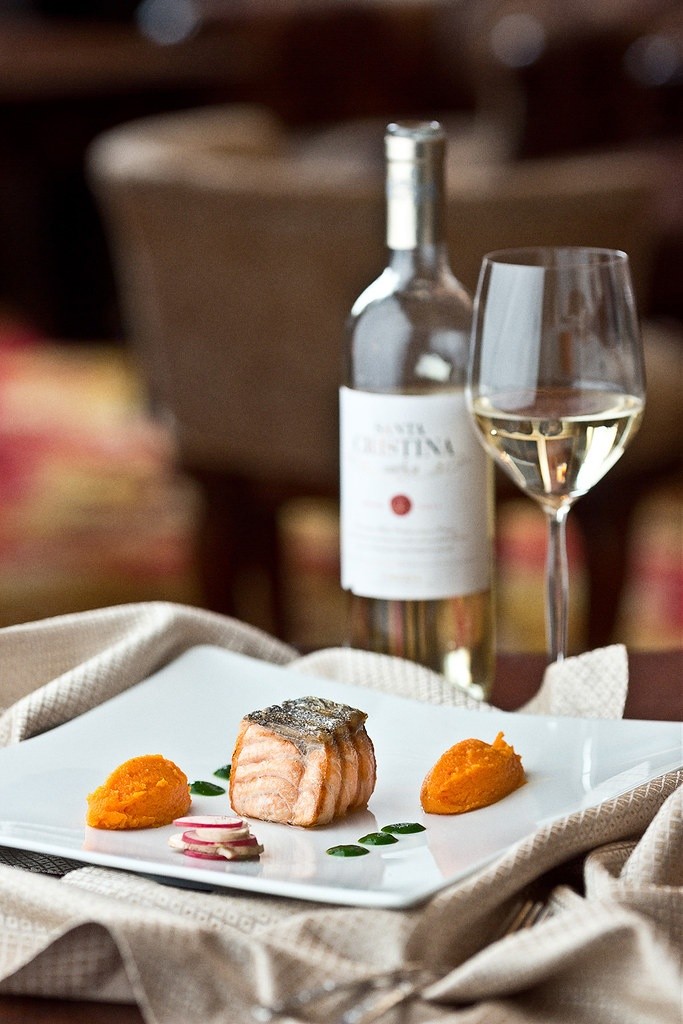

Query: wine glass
[464,246,648,665]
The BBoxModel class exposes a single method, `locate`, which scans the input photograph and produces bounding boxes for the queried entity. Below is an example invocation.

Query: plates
[0,643,683,910]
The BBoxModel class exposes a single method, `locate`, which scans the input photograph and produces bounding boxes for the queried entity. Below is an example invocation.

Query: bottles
[338,121,499,701]
